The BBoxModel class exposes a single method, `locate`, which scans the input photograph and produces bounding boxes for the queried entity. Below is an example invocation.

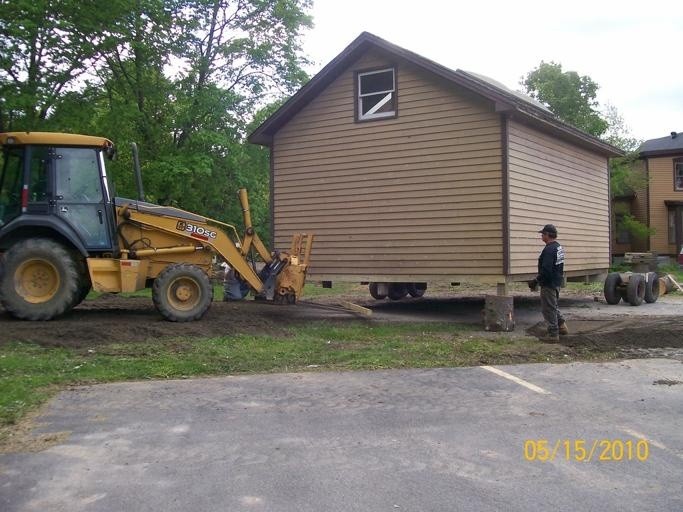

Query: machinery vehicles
[1,130,317,324]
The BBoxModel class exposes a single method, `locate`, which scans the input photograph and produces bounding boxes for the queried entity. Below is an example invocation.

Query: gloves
[528,279,538,289]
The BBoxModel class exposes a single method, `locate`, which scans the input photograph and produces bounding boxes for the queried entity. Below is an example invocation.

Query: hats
[537,223,558,238]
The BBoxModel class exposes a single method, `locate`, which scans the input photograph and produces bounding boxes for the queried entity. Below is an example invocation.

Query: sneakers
[541,333,561,343]
[559,322,569,334]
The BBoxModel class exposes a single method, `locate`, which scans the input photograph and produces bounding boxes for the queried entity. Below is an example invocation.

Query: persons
[527,224,569,343]
